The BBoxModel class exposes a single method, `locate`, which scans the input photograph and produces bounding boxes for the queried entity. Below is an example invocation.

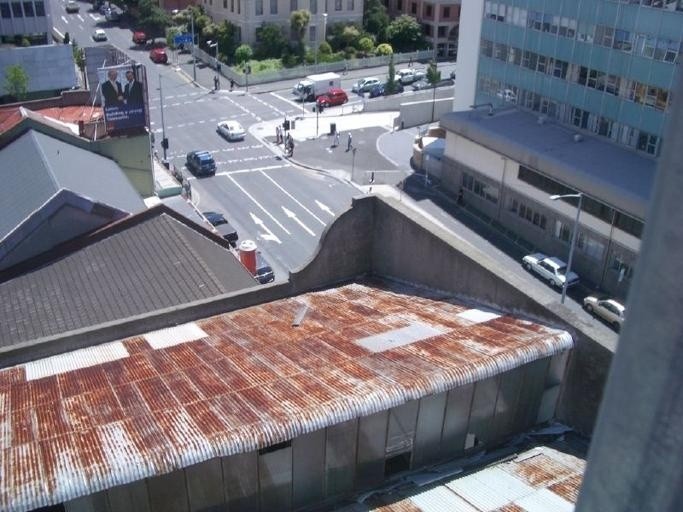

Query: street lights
[206,41,218,90]
[431,64,437,122]
[171,9,197,82]
[546,192,587,305]
[158,66,183,159]
[350,148,358,182]
[322,13,327,41]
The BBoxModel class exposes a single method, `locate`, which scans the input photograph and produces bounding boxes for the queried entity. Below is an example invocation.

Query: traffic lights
[247,66,251,73]
[163,138,168,149]
[242,69,245,73]
[285,121,290,130]
[290,120,295,129]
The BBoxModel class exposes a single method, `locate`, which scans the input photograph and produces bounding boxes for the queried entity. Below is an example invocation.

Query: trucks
[297,72,342,101]
[392,68,423,84]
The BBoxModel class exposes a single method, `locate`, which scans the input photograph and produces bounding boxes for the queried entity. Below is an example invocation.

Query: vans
[131,31,145,43]
[149,49,167,65]
[93,29,106,42]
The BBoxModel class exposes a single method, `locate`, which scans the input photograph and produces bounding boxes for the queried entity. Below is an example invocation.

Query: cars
[584,294,623,333]
[521,251,579,290]
[353,78,380,93]
[65,1,80,13]
[371,80,404,97]
[233,244,275,283]
[293,83,300,95]
[413,78,452,89]
[215,120,246,142]
[202,210,239,243]
[494,88,517,100]
[104,10,118,21]
[449,69,455,79]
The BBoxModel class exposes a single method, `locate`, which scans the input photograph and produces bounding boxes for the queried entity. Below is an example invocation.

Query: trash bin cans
[240,240,258,276]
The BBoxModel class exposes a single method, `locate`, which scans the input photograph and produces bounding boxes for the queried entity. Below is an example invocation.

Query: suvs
[186,150,216,177]
[317,87,347,107]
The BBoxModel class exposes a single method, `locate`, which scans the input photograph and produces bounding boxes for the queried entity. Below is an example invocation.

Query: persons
[230,79,235,90]
[101,71,125,106]
[408,56,413,67]
[121,71,143,106]
[275,125,294,157]
[214,75,219,90]
[343,63,349,74]
[333,128,340,148]
[346,132,353,152]
[457,187,464,206]
